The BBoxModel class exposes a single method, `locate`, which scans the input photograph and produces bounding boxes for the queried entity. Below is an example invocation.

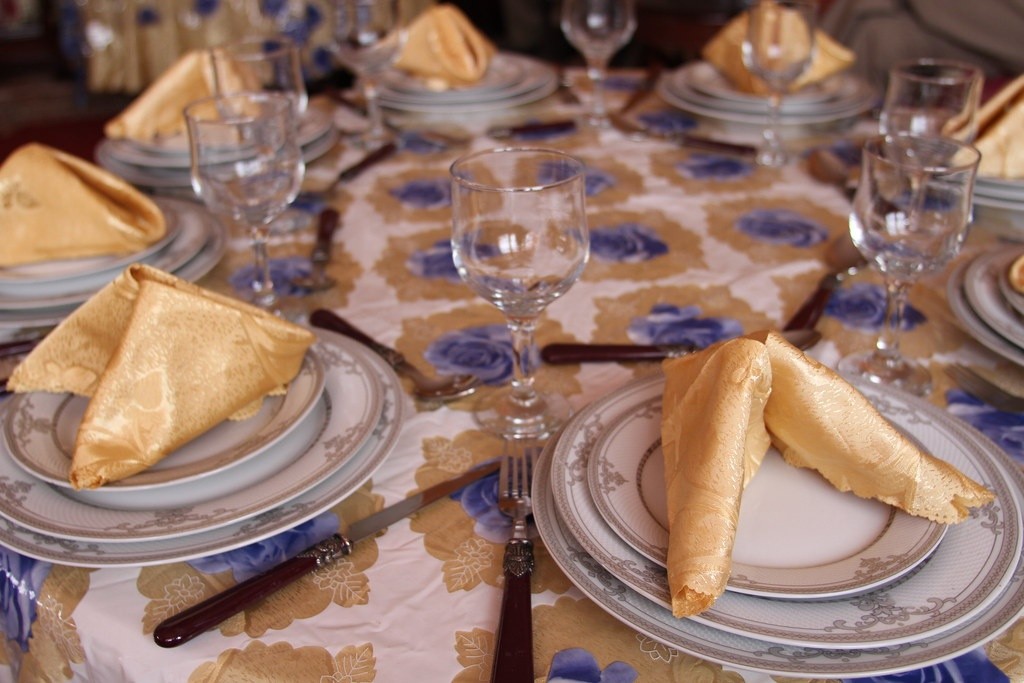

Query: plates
[0,325,405,570]
[95,106,339,185]
[0,194,227,344]
[376,49,559,115]
[948,245,1024,368]
[657,63,882,131]
[930,171,1024,217]
[532,369,1024,678]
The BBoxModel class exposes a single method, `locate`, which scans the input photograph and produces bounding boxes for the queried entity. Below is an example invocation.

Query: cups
[214,37,306,133]
[878,57,985,151]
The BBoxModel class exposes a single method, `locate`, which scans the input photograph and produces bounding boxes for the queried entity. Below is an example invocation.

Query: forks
[942,362,1023,414]
[491,442,537,683]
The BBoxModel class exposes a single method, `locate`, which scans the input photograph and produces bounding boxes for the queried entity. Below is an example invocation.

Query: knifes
[153,455,506,648]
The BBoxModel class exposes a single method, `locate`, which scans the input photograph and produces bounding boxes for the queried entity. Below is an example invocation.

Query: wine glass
[328,0,410,158]
[732,2,821,178]
[835,134,981,397]
[186,97,307,328]
[450,148,590,440]
[561,0,637,148]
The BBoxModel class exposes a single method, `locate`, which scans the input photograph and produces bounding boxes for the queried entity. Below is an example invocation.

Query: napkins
[104,46,258,146]
[660,327,989,618]
[4,263,314,490]
[700,0,860,94]
[944,72,1024,185]
[0,142,167,267]
[380,3,489,89]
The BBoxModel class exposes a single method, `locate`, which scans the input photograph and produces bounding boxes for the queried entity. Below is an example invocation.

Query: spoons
[310,309,482,401]
